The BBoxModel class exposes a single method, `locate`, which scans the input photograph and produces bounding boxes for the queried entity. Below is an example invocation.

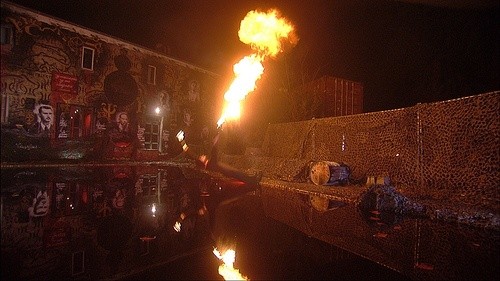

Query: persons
[181,130,256,184]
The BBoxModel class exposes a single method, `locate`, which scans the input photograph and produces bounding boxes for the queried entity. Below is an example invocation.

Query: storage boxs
[310,160,350,185]
